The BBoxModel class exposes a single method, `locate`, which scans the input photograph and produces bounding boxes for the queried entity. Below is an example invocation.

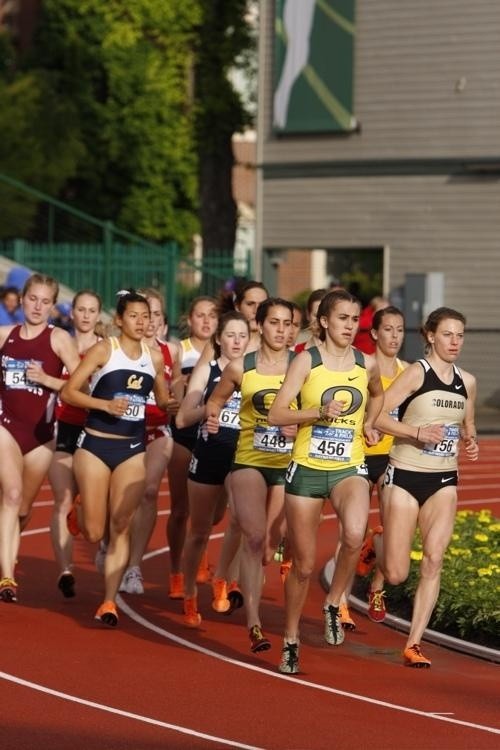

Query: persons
[0,273,479,674]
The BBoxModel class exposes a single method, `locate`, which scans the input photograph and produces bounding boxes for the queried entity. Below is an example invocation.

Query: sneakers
[400,644,433,667]
[321,599,355,646]
[209,577,244,616]
[94,601,119,627]
[196,548,210,584]
[57,570,75,598]
[95,539,143,594]
[247,624,271,652]
[67,494,81,536]
[368,589,388,622]
[354,526,383,577]
[168,572,202,628]
[274,544,293,583]
[0,577,18,601]
[277,639,301,676]
